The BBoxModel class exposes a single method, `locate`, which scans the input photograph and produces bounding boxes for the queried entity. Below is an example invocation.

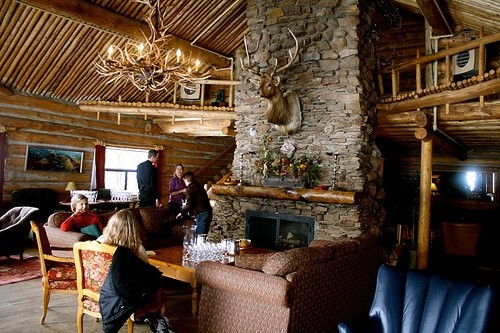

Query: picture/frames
[24,145,85,173]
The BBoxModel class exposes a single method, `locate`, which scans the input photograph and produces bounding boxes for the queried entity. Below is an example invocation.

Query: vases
[259,177,304,189]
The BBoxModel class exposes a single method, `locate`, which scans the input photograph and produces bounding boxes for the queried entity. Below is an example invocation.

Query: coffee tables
[145,244,276,319]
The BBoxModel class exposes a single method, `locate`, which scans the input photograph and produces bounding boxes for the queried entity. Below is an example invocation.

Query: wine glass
[70,189,98,204]
[109,189,132,202]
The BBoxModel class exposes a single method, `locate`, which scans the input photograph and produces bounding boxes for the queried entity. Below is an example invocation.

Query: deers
[239,27,306,126]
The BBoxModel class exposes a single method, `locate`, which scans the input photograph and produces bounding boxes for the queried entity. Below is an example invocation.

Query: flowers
[252,137,322,190]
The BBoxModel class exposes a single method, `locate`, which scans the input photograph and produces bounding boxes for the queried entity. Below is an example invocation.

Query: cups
[186,241,229,264]
[227,240,240,256]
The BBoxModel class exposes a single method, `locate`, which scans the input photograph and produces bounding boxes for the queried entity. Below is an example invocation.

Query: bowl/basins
[236,239,252,249]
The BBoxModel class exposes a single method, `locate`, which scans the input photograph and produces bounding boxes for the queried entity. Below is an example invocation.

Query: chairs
[11,188,58,248]
[30,220,78,324]
[73,240,166,333]
[0,206,40,261]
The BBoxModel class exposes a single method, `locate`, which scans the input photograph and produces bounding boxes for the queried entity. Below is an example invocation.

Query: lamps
[65,182,77,196]
[92,0,213,96]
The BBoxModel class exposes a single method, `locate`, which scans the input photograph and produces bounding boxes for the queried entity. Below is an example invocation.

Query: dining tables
[59,198,141,209]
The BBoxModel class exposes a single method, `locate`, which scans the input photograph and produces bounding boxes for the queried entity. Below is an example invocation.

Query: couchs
[338,263,494,333]
[195,234,384,333]
[43,206,196,258]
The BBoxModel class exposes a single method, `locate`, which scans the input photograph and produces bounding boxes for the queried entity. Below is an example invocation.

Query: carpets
[0,253,43,286]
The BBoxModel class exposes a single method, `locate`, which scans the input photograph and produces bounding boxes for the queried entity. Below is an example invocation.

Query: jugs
[181,224,197,262]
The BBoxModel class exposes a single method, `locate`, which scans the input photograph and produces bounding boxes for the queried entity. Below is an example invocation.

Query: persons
[137,149,162,208]
[168,164,187,224]
[95,208,170,329]
[60,194,104,236]
[176,170,213,241]
